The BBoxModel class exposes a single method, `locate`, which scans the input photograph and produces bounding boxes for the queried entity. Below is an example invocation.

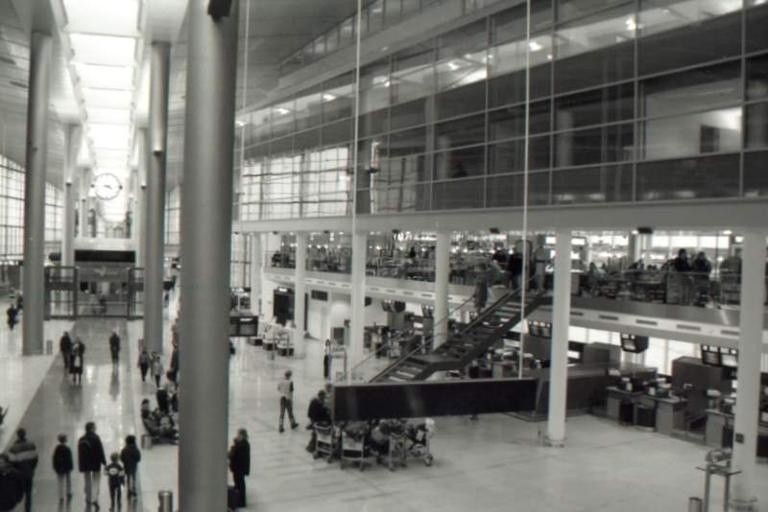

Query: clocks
[92,173,121,201]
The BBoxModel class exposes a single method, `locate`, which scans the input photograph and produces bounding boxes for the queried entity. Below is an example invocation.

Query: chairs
[313,417,434,472]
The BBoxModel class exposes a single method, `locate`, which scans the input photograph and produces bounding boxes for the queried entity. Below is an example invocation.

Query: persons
[409,247,416,258]
[428,246,435,279]
[60,330,165,388]
[156,368,177,415]
[6,303,18,331]
[492,244,522,289]
[323,339,332,377]
[0,406,10,424]
[228,428,251,507]
[140,397,179,447]
[0,420,179,512]
[306,389,331,454]
[323,383,334,416]
[13,290,22,312]
[277,369,299,433]
[675,246,741,308]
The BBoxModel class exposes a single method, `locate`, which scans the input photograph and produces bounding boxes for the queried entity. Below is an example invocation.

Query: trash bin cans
[159,492,172,512]
